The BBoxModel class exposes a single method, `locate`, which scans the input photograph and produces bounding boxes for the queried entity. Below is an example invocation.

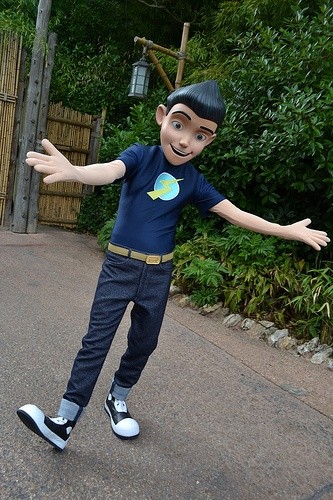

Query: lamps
[128,45,151,100]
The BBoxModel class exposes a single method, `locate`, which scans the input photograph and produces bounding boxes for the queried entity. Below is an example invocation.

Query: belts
[107,242,175,266]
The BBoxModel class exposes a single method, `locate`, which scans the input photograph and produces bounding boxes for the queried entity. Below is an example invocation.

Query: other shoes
[16,399,76,451]
[103,393,140,440]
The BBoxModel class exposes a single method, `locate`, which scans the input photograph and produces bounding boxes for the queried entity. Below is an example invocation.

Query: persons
[15,81,330,454]
